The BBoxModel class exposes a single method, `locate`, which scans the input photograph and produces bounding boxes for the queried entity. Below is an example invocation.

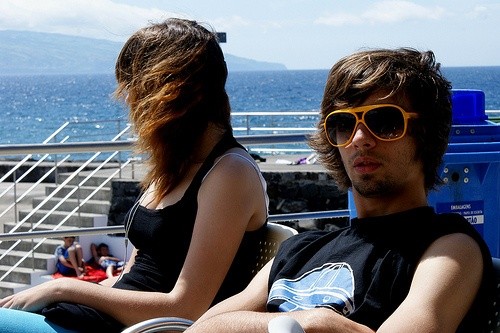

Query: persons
[182,46,499,333]
[52,235,87,280]
[0,18,269,333]
[90,241,129,280]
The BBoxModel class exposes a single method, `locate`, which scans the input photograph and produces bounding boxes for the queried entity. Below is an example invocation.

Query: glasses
[324,105,418,147]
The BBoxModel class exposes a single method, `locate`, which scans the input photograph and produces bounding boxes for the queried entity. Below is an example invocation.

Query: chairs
[123,224,299,333]
